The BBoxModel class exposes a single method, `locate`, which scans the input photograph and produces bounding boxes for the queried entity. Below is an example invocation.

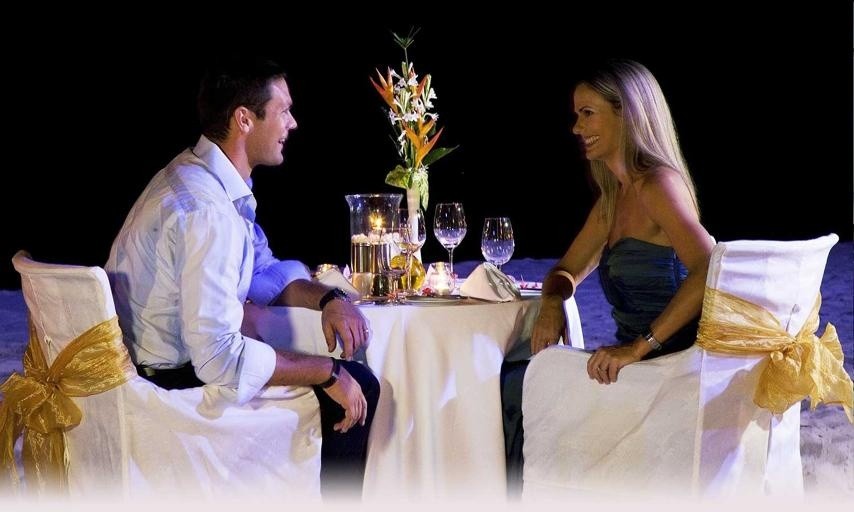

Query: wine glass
[433,203,467,272]
[481,217,516,271]
[393,208,428,296]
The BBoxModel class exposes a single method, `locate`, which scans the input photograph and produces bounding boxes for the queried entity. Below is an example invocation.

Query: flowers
[371,25,459,211]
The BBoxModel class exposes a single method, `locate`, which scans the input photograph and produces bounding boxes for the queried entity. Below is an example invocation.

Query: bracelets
[319,357,340,388]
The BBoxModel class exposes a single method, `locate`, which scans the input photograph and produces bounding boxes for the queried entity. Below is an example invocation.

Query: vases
[406,185,421,264]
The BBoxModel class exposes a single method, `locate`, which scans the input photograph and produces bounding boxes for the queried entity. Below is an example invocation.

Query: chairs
[521,231,841,497]
[11,249,322,497]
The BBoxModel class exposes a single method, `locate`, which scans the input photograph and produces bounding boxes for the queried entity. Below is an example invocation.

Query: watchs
[319,287,352,309]
[641,328,662,353]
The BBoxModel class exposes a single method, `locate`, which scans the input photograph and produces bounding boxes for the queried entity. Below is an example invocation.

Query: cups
[428,262,454,295]
[343,194,404,299]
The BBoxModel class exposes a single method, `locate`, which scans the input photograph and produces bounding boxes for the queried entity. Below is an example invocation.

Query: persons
[499,59,718,496]
[103,54,380,507]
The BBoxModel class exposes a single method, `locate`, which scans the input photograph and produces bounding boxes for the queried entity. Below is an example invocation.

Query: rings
[364,328,369,334]
[597,366,607,372]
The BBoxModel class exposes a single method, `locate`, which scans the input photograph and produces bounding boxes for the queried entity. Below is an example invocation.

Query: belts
[139,367,155,377]
